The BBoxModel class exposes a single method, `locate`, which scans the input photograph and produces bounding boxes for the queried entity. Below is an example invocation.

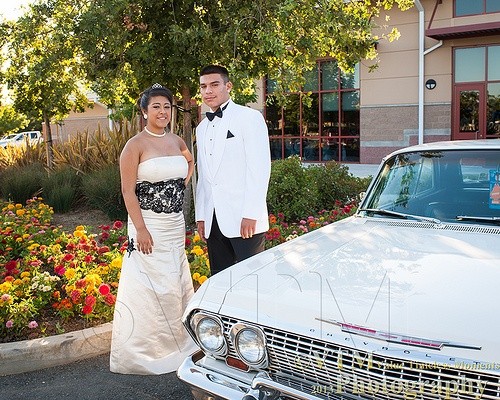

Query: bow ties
[206,102,229,121]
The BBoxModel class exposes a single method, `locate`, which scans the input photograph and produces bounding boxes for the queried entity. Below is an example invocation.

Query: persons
[110,86,195,375]
[195,65,271,277]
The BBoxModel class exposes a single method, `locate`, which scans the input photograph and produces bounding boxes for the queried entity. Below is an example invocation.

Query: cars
[0,131,41,150]
[176,138,500,400]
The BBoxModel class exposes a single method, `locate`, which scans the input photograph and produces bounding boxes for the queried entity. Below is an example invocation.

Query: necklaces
[144,126,167,137]
